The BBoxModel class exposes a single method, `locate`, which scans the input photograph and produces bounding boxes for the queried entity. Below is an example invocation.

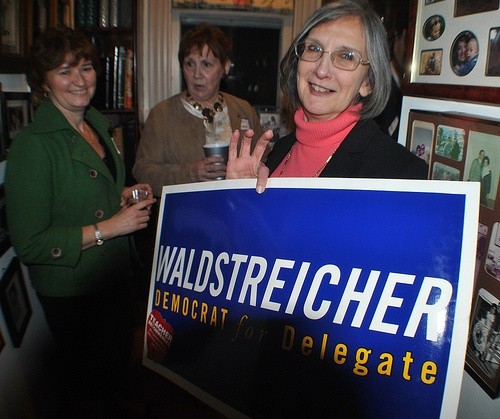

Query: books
[0,0,132,54]
[90,36,133,109]
[113,127,125,160]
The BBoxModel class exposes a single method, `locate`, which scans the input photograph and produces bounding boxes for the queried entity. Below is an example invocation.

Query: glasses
[294,39,369,72]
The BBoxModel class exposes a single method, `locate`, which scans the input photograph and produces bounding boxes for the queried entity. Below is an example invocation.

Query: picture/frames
[0,182,11,257]
[402,0,500,105]
[0,91,35,160]
[0,256,34,349]
[404,109,500,400]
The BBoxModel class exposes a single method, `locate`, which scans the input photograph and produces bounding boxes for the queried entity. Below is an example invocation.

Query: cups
[123,188,148,210]
[203,143,229,180]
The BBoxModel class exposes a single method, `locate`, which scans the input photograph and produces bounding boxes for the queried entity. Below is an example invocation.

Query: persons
[423,17,500,76]
[132,22,271,206]
[413,129,491,205]
[224,0,430,194]
[473,304,498,358]
[5,26,157,357]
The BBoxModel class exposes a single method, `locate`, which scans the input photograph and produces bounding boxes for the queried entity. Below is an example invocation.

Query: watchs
[93,223,105,246]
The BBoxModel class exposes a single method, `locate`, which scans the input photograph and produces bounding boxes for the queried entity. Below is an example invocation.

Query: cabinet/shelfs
[0,0,141,187]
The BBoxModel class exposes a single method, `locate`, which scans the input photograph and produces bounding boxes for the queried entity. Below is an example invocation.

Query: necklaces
[278,142,332,178]
[185,93,224,125]
